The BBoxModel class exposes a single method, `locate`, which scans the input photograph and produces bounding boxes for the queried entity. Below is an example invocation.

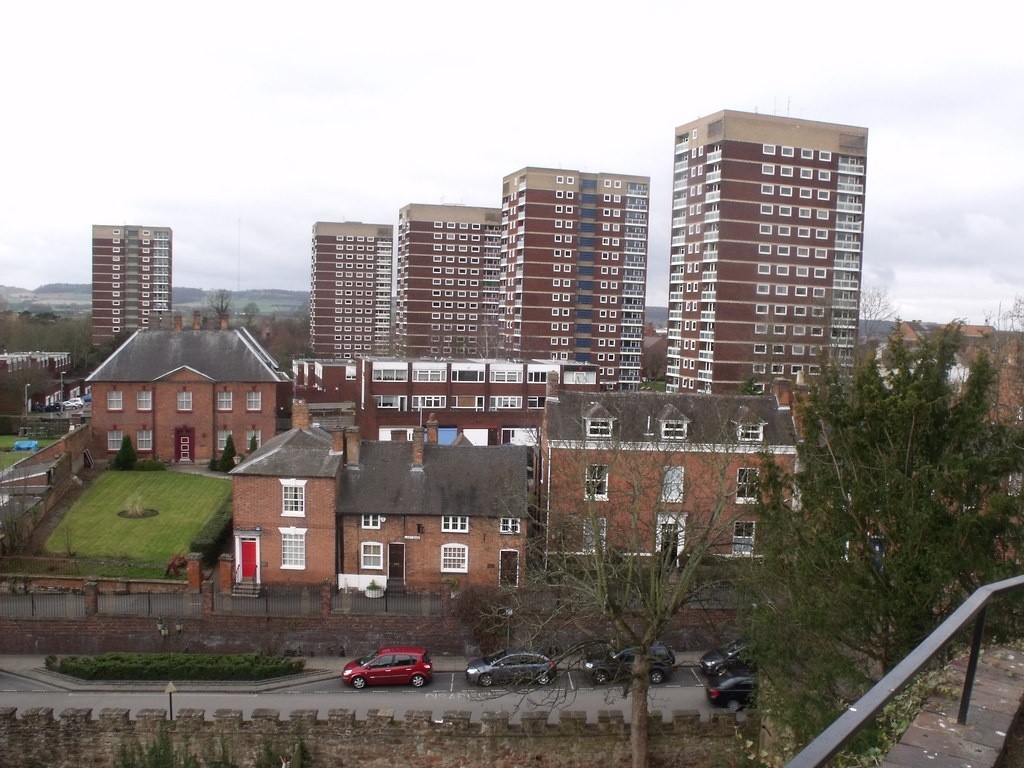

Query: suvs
[583,642,676,686]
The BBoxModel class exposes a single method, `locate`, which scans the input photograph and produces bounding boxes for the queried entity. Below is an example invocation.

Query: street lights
[25,384,30,417]
[61,372,66,411]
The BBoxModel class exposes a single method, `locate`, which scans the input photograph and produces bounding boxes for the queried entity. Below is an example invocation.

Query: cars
[706,660,758,712]
[699,639,746,677]
[44,398,84,412]
[341,646,432,690]
[465,647,556,688]
